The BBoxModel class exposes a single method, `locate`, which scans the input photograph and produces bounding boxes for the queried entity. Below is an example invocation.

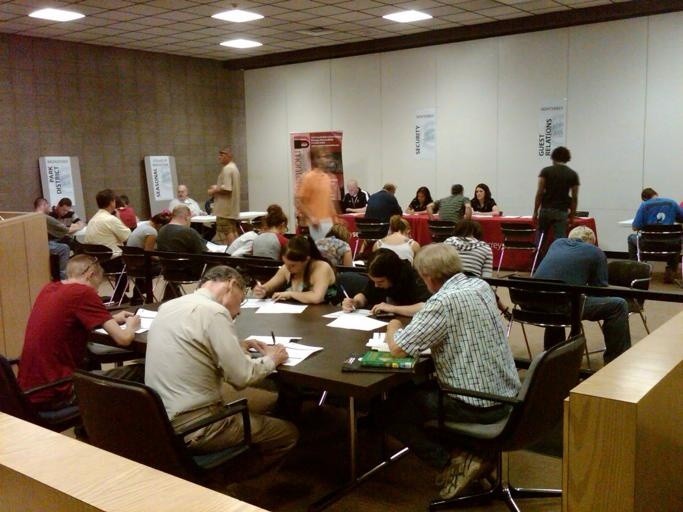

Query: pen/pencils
[134,308,139,316]
[271,331,275,345]
[254,278,262,287]
[340,284,356,311]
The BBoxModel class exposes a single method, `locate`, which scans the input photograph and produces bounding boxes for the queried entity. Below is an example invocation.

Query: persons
[443,219,493,278]
[427,184,482,239]
[342,247,433,318]
[406,186,438,215]
[471,183,499,215]
[365,183,403,219]
[531,226,631,366]
[17,254,144,442]
[33,143,370,304]
[627,188,683,283]
[144,266,300,470]
[531,146,580,272]
[386,242,522,500]
[372,215,421,267]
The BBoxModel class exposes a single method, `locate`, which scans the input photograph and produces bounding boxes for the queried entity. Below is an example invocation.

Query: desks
[87,295,433,512]
[164,210,272,235]
[296,214,596,271]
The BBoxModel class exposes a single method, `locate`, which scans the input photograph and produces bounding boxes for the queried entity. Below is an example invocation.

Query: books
[342,351,420,374]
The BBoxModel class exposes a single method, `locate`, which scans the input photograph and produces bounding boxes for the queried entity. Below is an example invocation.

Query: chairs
[428,219,457,242]
[0,355,101,433]
[635,225,683,286]
[71,368,273,483]
[429,337,584,512]
[506,276,608,370]
[583,259,652,354]
[335,273,370,305]
[351,217,391,260]
[48,242,286,305]
[498,220,546,276]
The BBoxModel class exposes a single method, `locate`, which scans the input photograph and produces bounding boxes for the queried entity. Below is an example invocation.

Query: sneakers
[441,452,483,499]
[435,467,451,485]
[664,269,676,281]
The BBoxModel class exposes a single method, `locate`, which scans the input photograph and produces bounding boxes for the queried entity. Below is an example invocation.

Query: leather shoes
[99,289,153,306]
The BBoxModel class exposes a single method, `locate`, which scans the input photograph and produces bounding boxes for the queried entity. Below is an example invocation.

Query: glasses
[82,256,99,275]
